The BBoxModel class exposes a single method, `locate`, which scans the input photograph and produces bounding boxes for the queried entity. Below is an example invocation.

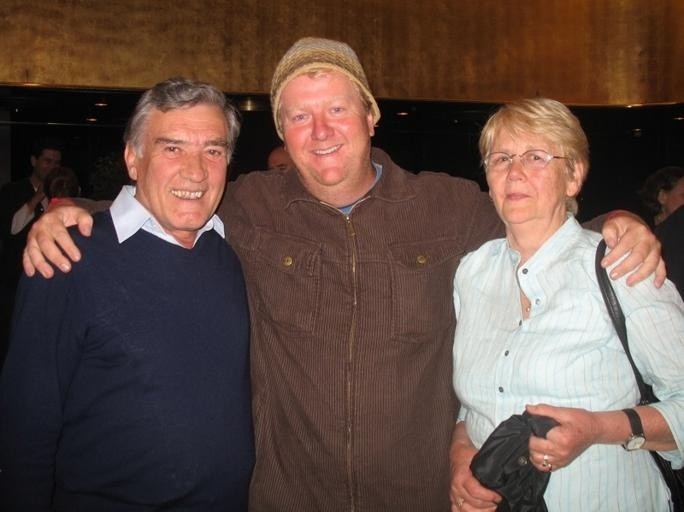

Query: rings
[455,497,464,508]
[548,464,553,472]
[542,454,548,467]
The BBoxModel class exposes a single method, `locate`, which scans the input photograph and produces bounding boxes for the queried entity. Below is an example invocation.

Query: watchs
[621,407,646,452]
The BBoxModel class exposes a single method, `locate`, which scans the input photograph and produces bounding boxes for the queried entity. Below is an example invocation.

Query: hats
[270,36,382,143]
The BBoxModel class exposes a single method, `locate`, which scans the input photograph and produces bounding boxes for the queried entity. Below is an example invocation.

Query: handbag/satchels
[637,385,684,512]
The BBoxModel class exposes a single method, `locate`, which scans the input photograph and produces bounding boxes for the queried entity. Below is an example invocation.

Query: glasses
[483,149,568,172]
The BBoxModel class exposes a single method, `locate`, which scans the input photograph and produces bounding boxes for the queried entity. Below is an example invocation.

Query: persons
[633,166,683,233]
[20,34,668,511]
[0,75,255,511]
[1,141,83,278]
[267,145,294,172]
[447,96,684,512]
